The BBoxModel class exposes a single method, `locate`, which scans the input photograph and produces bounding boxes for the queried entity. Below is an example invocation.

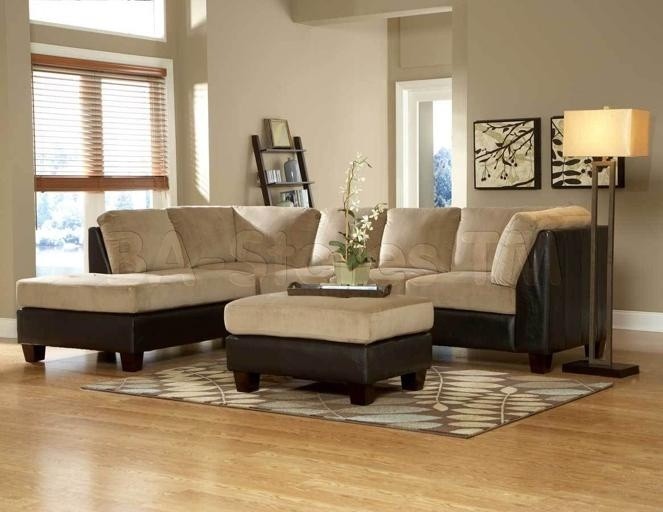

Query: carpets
[82,358,615,441]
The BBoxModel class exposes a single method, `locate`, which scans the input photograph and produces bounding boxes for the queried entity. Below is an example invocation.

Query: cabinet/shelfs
[252,136,314,205]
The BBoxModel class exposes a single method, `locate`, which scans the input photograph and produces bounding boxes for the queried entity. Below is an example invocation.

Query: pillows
[97,205,592,288]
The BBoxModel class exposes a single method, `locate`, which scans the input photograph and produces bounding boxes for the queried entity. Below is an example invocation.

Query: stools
[224,291,436,405]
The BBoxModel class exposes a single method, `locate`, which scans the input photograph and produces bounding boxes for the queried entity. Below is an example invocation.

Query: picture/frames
[474,117,625,190]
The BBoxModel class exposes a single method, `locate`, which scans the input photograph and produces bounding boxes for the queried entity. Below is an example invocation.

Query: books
[254,169,283,187]
[279,190,308,210]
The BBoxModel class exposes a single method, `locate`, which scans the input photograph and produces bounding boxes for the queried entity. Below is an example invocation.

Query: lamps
[563,109,651,377]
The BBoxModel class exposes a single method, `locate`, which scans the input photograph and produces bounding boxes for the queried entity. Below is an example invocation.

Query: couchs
[15,226,608,374]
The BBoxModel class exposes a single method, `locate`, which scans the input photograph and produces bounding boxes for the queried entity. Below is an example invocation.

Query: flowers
[328,151,388,268]
